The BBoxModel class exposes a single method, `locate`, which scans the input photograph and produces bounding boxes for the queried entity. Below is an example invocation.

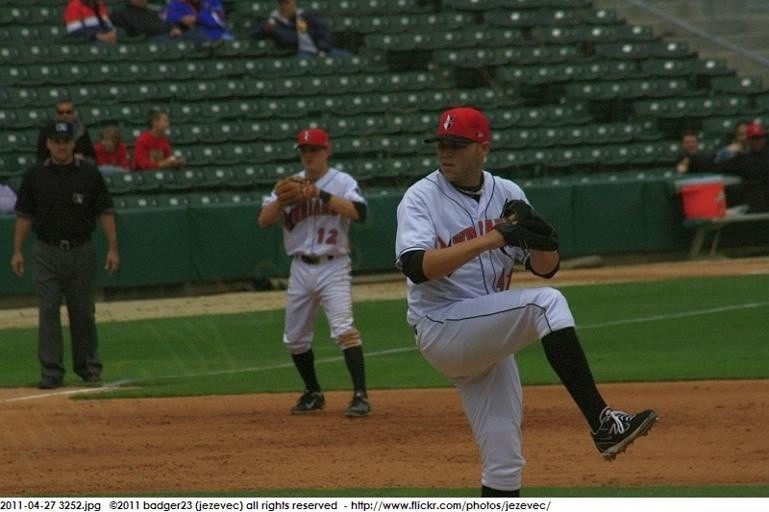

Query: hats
[745,121,766,139]
[293,128,331,152]
[45,121,74,143]
[423,106,491,147]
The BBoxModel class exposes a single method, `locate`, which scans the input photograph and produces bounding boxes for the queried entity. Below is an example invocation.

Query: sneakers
[343,392,373,419]
[588,404,659,463]
[289,388,328,414]
[36,378,64,389]
[82,376,103,384]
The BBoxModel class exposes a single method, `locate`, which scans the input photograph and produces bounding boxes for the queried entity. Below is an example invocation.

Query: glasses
[57,109,76,115]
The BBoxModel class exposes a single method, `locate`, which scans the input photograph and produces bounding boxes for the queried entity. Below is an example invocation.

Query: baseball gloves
[492,199,559,252]
[274,175,316,208]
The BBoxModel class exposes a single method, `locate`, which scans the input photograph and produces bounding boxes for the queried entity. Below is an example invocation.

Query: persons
[396,106,661,495]
[10,118,121,386]
[63,2,353,59]
[713,120,753,164]
[36,98,98,166]
[669,132,718,176]
[702,122,769,214]
[257,126,374,419]
[129,108,188,170]
[93,122,128,171]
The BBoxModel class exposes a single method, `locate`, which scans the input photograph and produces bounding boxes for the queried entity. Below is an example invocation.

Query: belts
[38,234,93,251]
[295,254,337,265]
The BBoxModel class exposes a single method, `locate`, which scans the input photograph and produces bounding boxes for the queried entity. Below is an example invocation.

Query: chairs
[0,0,769,273]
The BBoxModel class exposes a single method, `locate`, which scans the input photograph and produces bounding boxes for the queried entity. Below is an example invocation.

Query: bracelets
[318,188,333,205]
